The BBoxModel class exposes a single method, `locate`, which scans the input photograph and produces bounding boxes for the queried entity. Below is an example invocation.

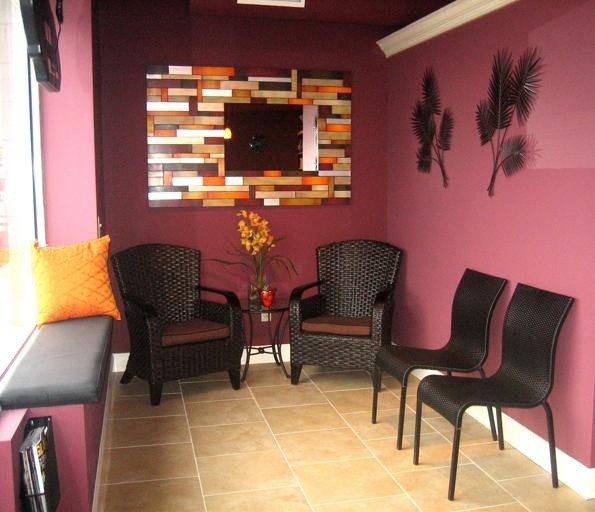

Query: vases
[246,282,265,308]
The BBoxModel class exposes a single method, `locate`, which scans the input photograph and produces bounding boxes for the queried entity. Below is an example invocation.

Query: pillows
[30,235,122,325]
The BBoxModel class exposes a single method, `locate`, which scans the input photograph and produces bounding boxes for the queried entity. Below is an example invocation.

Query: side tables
[238,298,291,382]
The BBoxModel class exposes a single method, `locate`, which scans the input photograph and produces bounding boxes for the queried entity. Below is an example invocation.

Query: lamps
[223,127,233,141]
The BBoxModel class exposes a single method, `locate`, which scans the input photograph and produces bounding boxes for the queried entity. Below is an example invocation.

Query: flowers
[202,207,299,309]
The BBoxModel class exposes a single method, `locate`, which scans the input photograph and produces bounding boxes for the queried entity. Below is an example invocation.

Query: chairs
[289,239,405,392]
[414,282,575,501]
[370,268,509,450]
[111,242,243,407]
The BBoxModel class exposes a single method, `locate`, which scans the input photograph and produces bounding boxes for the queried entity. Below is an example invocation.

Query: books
[19,425,51,512]
